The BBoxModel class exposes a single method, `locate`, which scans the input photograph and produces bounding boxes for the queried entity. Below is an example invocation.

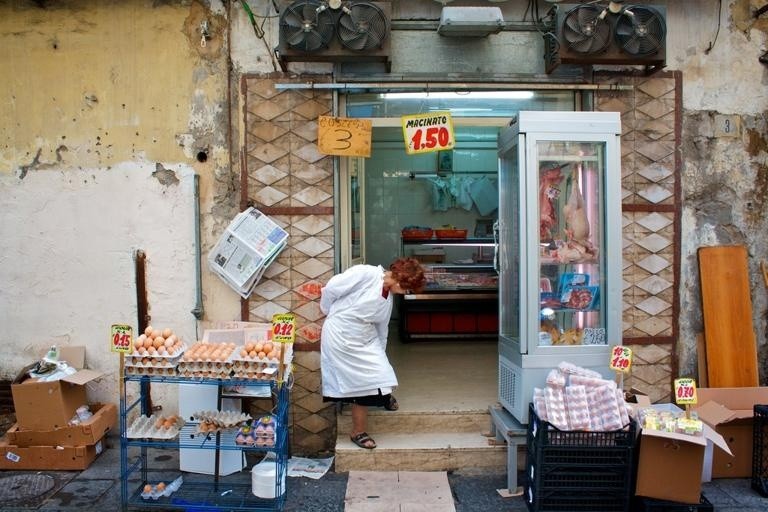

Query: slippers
[349,431,376,450]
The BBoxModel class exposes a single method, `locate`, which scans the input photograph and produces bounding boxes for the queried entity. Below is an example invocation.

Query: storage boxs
[633,407,735,504]
[10,344,107,430]
[7,403,117,445]
[652,402,715,483]
[623,386,651,411]
[0,435,108,470]
[685,385,766,479]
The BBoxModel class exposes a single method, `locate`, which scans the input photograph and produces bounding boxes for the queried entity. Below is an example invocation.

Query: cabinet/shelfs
[120,371,289,510]
[396,238,499,341]
[493,108,627,426]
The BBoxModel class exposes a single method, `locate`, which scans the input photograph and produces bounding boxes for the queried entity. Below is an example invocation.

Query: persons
[316,255,428,450]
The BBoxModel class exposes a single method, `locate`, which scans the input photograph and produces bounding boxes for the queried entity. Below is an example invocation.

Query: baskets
[751,403,767,499]
[524,401,641,510]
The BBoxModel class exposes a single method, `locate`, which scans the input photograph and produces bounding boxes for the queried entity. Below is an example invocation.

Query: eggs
[156,416,177,429]
[130,326,280,380]
[199,422,217,434]
[237,416,275,449]
[144,484,152,493]
[157,482,166,490]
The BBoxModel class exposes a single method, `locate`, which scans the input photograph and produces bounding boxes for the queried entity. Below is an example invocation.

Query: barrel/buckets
[251,461,286,499]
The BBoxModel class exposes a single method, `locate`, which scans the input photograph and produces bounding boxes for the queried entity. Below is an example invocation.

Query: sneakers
[383,394,399,411]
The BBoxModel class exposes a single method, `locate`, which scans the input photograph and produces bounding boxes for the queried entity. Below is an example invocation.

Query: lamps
[435,6,506,41]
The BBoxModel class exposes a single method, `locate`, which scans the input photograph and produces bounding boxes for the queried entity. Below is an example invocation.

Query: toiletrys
[68,404,94,426]
[37,367,77,383]
